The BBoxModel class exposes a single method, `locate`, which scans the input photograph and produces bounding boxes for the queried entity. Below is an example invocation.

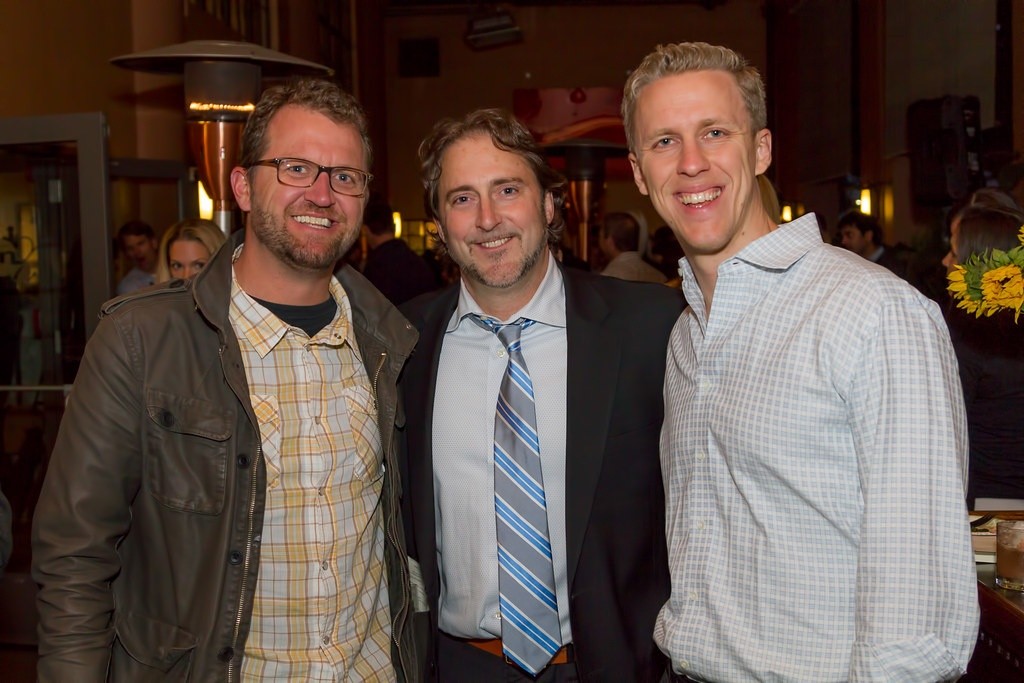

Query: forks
[971,512,997,528]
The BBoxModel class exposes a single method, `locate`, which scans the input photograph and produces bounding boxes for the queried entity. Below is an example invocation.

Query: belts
[465,636,570,665]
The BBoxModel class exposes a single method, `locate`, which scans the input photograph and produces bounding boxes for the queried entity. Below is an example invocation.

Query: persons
[351,192,460,304]
[115,219,162,295]
[549,207,685,290]
[30,74,424,683]
[156,217,227,283]
[392,107,687,683]
[620,43,981,683]
[835,191,1024,509]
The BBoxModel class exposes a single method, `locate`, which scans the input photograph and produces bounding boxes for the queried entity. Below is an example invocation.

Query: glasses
[245,157,373,196]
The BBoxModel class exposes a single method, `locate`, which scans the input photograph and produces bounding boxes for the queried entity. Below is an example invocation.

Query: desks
[957,510,1024,683]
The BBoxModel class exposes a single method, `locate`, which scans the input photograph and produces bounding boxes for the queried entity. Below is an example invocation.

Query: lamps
[463,11,523,52]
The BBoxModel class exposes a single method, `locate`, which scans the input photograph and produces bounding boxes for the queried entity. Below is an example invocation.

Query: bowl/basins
[969,515,1004,551]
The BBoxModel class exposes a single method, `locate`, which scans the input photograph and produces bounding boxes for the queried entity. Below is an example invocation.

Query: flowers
[946,223,1024,324]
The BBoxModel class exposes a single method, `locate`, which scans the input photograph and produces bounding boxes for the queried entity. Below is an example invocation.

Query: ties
[468,313,563,678]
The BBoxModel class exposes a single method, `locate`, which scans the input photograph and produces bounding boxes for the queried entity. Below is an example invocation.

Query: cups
[997,521,1024,591]
